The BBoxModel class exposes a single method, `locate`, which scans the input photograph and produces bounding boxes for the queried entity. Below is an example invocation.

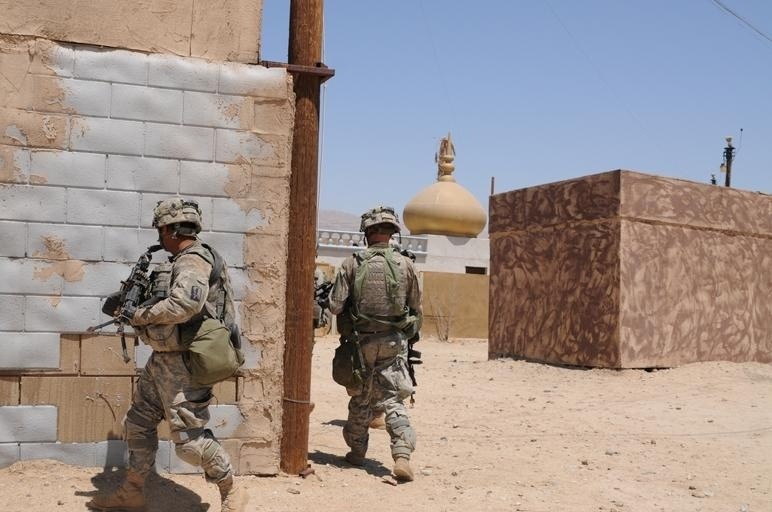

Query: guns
[85,245,167,361]
[315,277,334,324]
[409,333,422,406]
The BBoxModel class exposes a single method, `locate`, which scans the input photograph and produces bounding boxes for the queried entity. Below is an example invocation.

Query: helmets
[152,199,202,234]
[360,207,401,234]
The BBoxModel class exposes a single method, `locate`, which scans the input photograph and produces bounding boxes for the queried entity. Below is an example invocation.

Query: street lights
[720,135,736,185]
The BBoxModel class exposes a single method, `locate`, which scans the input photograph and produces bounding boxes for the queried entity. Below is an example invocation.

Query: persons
[91,197,246,512]
[369,240,401,431]
[329,206,422,482]
[313,239,328,351]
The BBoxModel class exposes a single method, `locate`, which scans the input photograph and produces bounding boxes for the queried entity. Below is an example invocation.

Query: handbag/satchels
[332,339,367,388]
[180,317,241,389]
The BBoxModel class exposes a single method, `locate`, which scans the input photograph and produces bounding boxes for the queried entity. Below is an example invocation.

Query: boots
[394,456,415,482]
[91,467,146,511]
[345,447,367,467]
[205,465,249,512]
